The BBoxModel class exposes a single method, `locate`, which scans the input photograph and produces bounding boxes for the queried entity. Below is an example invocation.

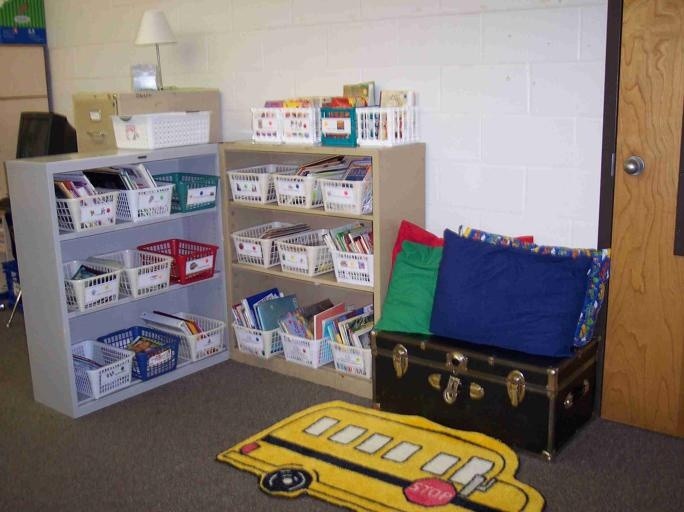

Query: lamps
[133,9,179,92]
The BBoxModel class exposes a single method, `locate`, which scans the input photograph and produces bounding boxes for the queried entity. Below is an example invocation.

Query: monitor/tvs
[16,111,78,159]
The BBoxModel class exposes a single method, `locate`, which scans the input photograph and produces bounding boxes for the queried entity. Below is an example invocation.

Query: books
[277,298,364,340]
[323,221,374,255]
[362,89,415,138]
[258,223,312,240]
[53,171,98,199]
[327,310,374,374]
[127,336,164,353]
[257,96,331,136]
[72,354,101,369]
[294,155,346,176]
[84,164,157,189]
[341,156,373,181]
[232,287,298,331]
[139,311,218,355]
[322,81,375,137]
[64,264,116,307]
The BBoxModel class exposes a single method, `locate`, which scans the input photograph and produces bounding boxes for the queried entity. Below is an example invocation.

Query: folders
[254,293,298,329]
[139,311,190,338]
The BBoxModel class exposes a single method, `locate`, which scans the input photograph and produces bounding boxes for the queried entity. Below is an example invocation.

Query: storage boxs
[371,329,602,463]
[69,90,223,154]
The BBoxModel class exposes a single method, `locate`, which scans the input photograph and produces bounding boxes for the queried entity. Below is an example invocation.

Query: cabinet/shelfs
[5,142,230,421]
[220,141,428,401]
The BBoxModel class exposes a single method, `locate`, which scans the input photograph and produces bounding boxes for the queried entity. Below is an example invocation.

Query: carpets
[214,399,546,511]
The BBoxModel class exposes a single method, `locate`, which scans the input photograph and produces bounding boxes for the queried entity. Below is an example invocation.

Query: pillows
[370,220,612,358]
